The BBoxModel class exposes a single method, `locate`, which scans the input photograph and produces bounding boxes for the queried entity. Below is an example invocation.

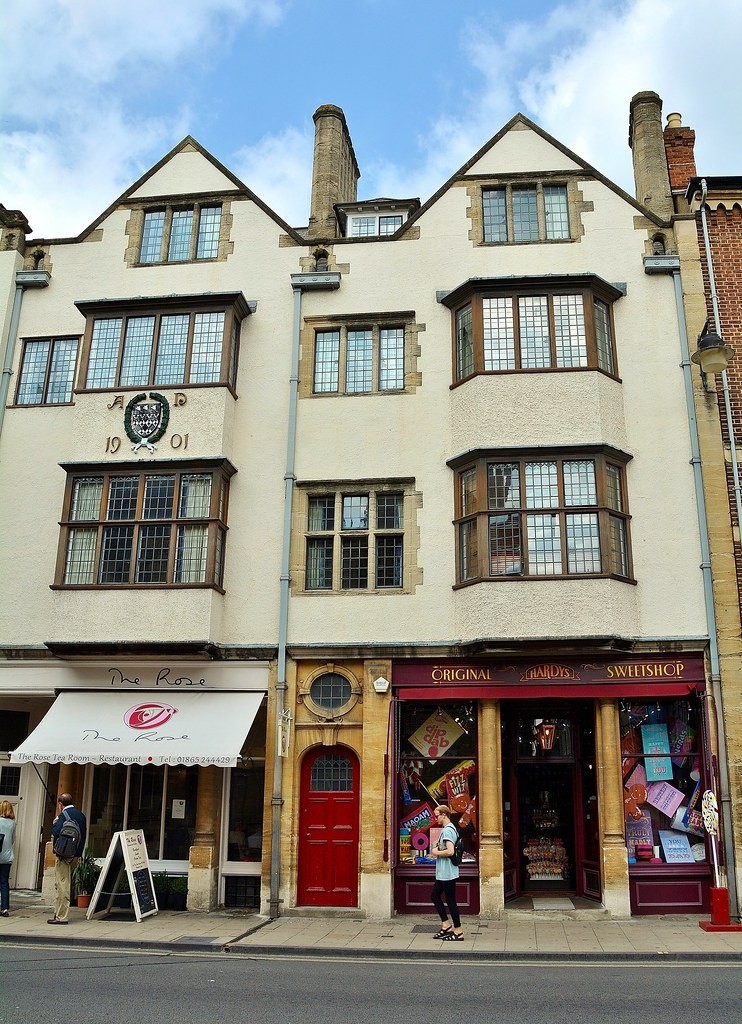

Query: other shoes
[0,910,10,917]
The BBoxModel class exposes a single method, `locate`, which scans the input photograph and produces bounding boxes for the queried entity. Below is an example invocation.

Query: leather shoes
[47,918,68,925]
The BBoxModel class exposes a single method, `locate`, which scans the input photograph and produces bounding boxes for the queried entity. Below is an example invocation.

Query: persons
[0,801,16,917]
[47,792,87,925]
[431,805,464,941]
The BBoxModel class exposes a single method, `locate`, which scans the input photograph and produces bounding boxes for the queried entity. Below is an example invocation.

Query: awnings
[7,692,266,807]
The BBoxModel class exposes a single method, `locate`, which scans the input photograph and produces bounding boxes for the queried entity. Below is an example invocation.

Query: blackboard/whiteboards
[86,830,156,915]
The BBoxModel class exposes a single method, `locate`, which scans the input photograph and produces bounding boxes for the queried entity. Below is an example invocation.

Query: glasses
[435,813,442,818]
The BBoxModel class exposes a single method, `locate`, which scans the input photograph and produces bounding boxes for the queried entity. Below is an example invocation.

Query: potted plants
[71,847,102,907]
[153,871,171,910]
[170,877,187,911]
[117,870,131,908]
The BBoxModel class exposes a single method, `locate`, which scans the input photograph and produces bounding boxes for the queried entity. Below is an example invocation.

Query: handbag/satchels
[0,834,5,853]
[52,810,81,865]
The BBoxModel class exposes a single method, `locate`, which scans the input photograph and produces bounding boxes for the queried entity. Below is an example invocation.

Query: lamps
[691,318,735,394]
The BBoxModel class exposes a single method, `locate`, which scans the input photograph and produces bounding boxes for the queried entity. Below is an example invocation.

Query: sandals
[432,925,453,939]
[441,931,465,941]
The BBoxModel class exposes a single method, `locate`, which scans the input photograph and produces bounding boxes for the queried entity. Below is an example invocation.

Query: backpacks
[436,826,464,867]
